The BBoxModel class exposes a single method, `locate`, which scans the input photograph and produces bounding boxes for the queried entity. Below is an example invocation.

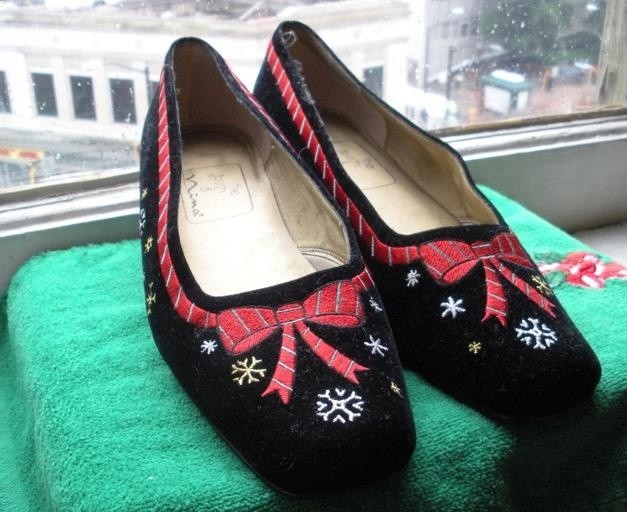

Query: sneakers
[141,37,416,499]
[254,21,602,424]
